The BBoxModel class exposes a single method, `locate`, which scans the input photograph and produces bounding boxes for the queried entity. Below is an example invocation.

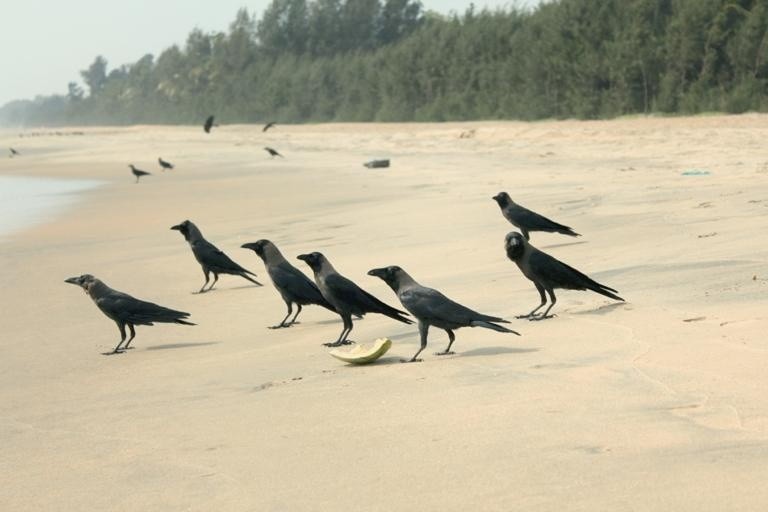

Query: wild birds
[128,163,149,183]
[169,219,264,294]
[157,157,174,171]
[240,239,366,329]
[262,121,283,157]
[203,115,218,134]
[491,191,583,242]
[296,252,417,347]
[504,231,625,322]
[367,265,521,363]
[64,274,199,355]
